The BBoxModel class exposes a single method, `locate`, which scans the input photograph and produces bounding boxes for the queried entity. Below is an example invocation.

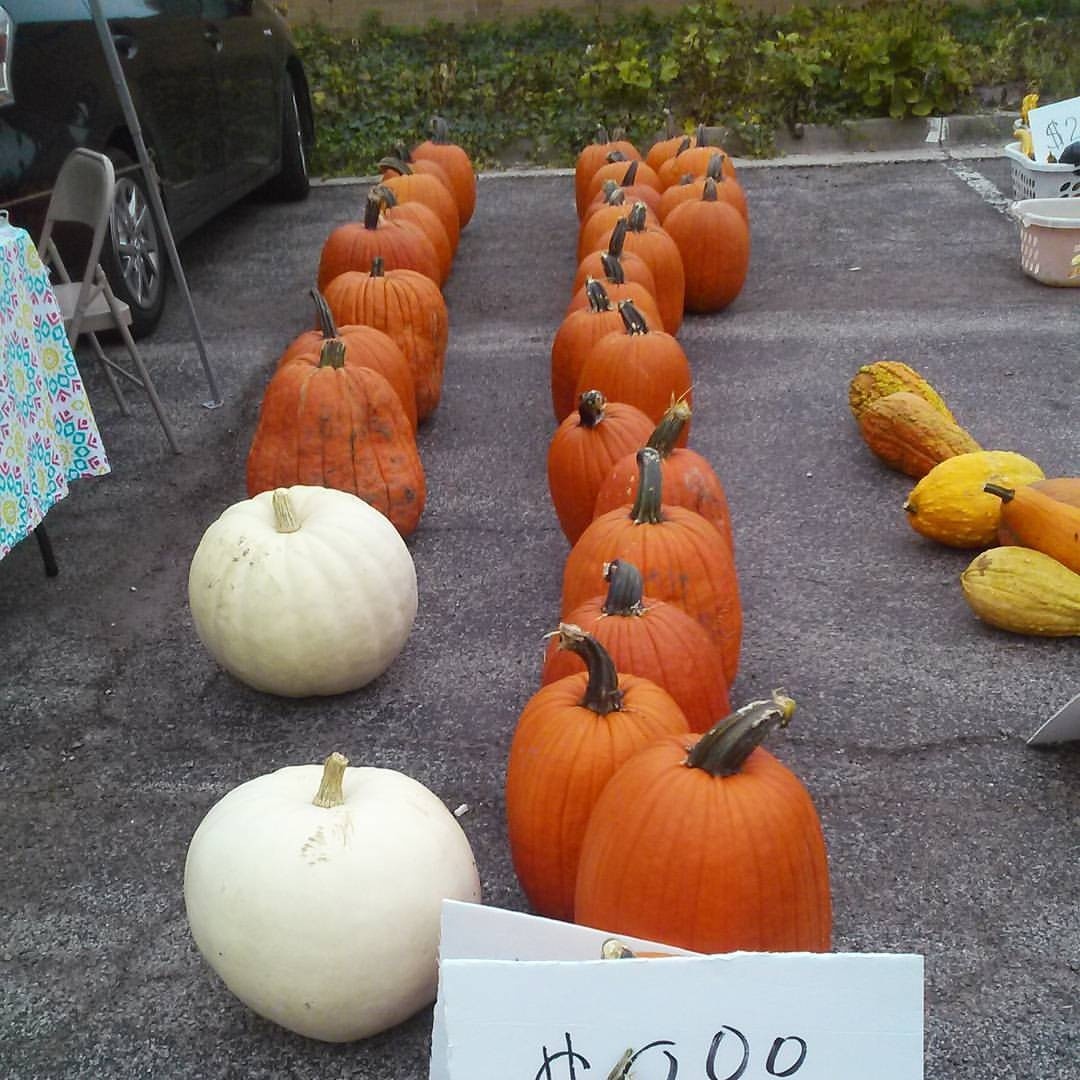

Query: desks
[0,207,113,582]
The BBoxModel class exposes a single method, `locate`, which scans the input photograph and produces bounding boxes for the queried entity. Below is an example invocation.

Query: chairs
[35,146,183,456]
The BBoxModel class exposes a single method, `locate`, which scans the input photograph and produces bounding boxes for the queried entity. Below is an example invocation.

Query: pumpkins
[185,108,1080,1049]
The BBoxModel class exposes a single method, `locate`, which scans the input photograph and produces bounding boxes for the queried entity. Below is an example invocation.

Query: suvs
[0,0,316,349]
